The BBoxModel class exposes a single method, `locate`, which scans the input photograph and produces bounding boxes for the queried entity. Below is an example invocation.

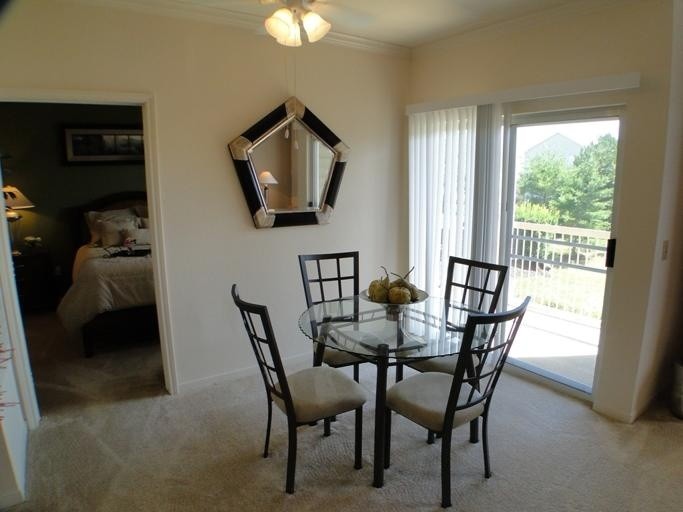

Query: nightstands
[14,245,54,317]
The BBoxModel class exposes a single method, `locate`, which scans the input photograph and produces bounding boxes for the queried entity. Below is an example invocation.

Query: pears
[368,275,418,303]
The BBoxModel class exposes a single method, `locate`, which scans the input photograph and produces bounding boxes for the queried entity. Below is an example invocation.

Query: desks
[298,292,509,474]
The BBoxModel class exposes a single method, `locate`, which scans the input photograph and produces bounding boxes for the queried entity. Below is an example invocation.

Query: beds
[55,191,160,358]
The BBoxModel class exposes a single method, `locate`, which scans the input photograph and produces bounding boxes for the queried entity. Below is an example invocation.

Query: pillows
[84,205,150,249]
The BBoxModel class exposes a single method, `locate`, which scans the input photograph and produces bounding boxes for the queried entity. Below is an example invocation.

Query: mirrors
[228,98,351,229]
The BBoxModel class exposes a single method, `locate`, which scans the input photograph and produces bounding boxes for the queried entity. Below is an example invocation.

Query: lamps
[259,0,331,47]
[284,121,302,150]
[0,151,14,177]
[3,185,36,256]
[258,170,278,208]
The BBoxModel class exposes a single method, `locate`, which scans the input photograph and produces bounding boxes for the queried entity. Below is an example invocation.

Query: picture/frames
[64,127,145,164]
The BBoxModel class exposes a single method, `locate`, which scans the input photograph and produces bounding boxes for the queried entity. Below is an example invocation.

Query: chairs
[380,295,532,508]
[296,251,386,440]
[232,283,366,497]
[395,252,509,444]
